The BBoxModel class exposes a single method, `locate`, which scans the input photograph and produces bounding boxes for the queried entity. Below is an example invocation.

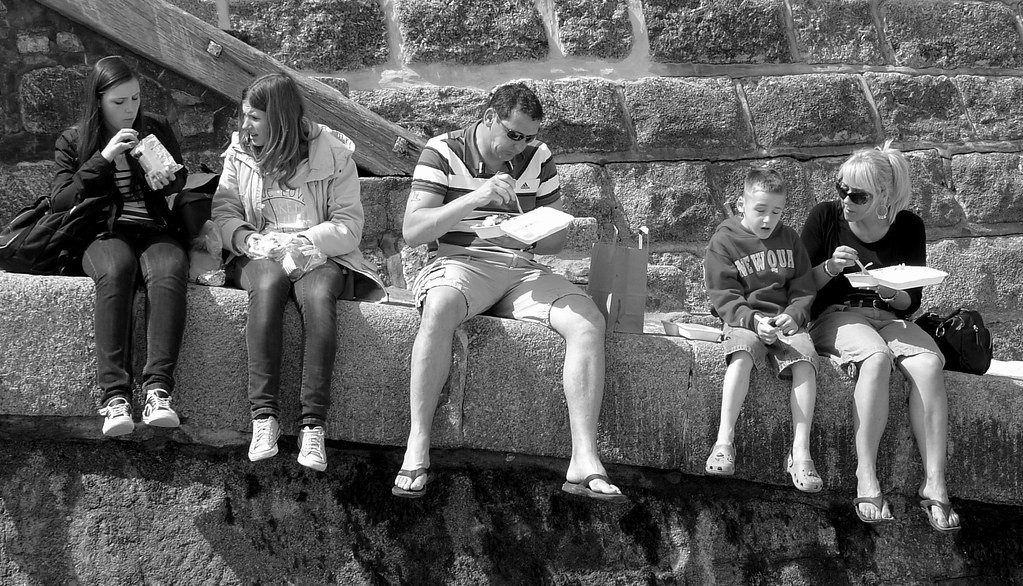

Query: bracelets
[823,259,839,277]
[519,242,537,253]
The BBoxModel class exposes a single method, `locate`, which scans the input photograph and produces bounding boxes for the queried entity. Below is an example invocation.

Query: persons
[211,73,388,471]
[801,141,961,532]
[704,168,822,493]
[392,82,627,502]
[51,56,189,436]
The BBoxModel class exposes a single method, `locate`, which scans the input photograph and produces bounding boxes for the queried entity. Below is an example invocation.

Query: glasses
[836,177,871,205]
[494,110,536,143]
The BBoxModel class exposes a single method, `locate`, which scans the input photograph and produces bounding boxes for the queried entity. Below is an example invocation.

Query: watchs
[879,291,899,303]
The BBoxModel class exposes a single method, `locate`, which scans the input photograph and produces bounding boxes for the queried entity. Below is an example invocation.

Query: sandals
[705,441,736,474]
[784,450,823,492]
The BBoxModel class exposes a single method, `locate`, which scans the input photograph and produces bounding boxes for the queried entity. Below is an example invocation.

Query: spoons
[513,193,523,214]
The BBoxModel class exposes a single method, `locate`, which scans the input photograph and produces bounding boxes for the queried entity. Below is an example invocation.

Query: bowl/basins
[469,206,576,245]
[843,264,948,290]
[662,320,723,342]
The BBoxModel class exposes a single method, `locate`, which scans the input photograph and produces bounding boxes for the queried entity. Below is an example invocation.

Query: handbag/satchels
[590,226,650,334]
[187,220,225,285]
[257,232,328,279]
[915,307,993,374]
[168,163,219,237]
[0,194,108,277]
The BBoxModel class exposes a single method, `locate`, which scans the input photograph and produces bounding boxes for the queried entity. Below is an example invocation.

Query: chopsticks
[754,314,790,342]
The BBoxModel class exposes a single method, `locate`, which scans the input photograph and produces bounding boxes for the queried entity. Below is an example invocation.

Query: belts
[850,300,891,311]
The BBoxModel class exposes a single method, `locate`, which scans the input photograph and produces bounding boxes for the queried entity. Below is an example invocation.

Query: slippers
[915,495,961,534]
[850,493,895,523]
[391,465,431,498]
[562,474,628,503]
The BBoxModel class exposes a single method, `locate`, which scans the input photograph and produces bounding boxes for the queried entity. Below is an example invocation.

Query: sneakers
[98,397,133,436]
[248,415,281,461]
[297,426,328,471]
[141,388,180,428]
